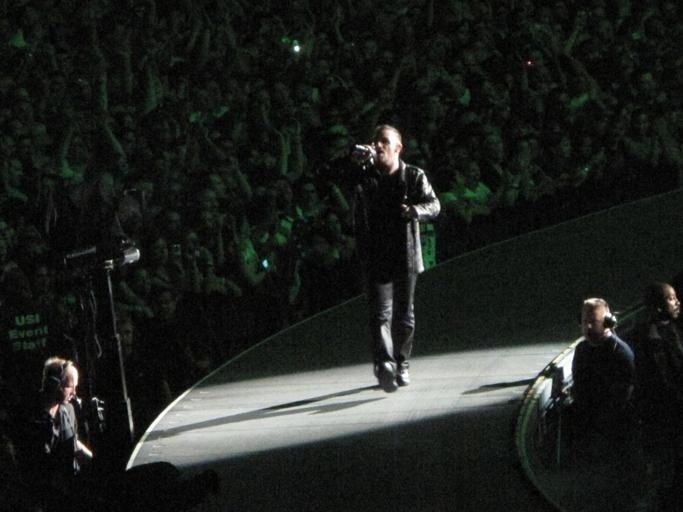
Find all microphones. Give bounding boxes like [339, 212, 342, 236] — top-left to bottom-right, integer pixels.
[610, 328, 618, 351]
[354, 143, 379, 156]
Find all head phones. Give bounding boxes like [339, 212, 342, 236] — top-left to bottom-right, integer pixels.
[576, 297, 616, 328]
[43, 360, 74, 395]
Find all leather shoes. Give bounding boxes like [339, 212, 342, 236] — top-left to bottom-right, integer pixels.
[374, 361, 411, 393]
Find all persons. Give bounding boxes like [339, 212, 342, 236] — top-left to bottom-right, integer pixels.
[347, 123, 443, 393]
[550, 281, 683, 512]
[343, 1, 682, 281]
[1, 1, 343, 511]
